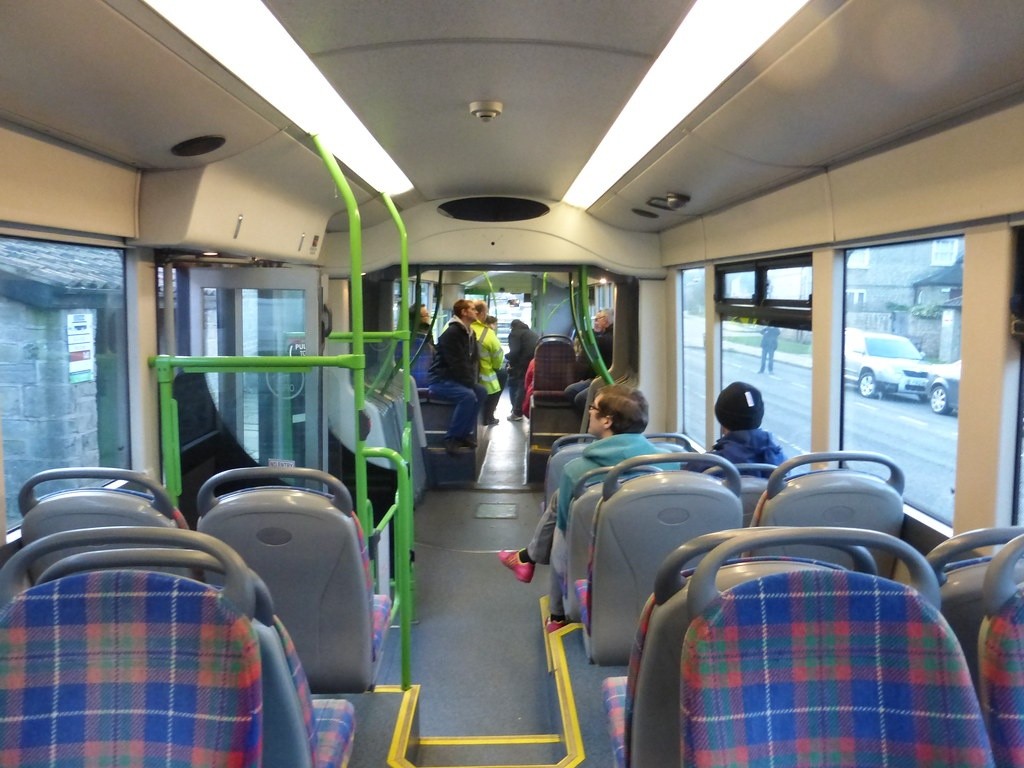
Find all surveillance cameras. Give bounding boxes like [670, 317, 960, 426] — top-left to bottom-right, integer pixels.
[666, 193, 690, 209]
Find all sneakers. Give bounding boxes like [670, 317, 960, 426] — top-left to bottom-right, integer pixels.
[546, 616, 565, 633]
[499, 548, 535, 583]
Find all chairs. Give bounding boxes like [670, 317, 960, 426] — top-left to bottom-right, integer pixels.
[533, 335, 577, 397]
[396, 331, 437, 396]
[545, 433, 1024, 768]
[0, 466, 393, 768]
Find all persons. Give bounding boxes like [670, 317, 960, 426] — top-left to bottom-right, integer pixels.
[758, 326, 780, 373]
[498, 381, 787, 633]
[409, 300, 539, 450]
[564, 309, 613, 412]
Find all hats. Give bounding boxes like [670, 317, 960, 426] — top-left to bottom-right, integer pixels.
[715, 382, 764, 431]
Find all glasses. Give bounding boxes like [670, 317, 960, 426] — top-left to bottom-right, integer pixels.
[595, 316, 605, 321]
[589, 402, 599, 411]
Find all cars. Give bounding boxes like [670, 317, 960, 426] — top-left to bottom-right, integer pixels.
[487, 298, 522, 342]
[843, 327, 931, 404]
[926, 361, 961, 414]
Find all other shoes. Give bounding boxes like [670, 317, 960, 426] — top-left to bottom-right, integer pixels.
[507, 412, 523, 421]
[442, 440, 456, 453]
[459, 438, 478, 447]
[487, 419, 499, 424]
